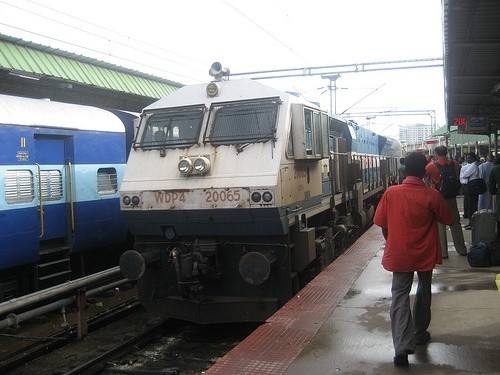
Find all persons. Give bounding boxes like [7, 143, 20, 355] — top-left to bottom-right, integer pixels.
[398, 158, 406, 184]
[373, 152, 454, 366]
[424, 146, 467, 258]
[450, 152, 500, 229]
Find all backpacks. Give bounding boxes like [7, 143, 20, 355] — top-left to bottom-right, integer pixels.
[434, 161, 462, 197]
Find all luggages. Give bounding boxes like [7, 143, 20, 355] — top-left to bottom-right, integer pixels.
[471, 208, 496, 244]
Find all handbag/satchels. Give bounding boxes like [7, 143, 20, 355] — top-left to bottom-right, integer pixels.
[466, 241, 499, 267]
[461, 179, 487, 195]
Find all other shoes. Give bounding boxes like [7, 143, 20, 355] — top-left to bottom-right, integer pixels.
[464, 224, 472, 230]
[393, 354, 409, 365]
[414, 332, 430, 344]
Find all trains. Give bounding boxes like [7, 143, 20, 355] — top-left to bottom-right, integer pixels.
[0, 93, 128, 314]
[119, 62, 408, 324]
